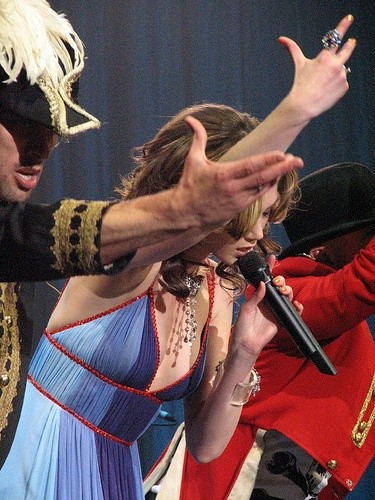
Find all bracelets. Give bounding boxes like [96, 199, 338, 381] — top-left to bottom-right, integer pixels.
[212, 358, 261, 406]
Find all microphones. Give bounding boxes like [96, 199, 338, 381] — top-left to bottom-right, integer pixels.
[236, 250, 337, 376]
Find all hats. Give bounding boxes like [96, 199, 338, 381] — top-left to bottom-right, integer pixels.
[277, 162, 375, 261]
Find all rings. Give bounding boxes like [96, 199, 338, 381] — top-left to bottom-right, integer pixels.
[321, 29, 342, 50]
[345, 67, 352, 76]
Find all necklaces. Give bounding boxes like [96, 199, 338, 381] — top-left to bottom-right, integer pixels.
[181, 275, 205, 355]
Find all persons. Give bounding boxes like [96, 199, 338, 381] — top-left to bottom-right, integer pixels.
[141, 162, 375, 500]
[0, 15, 355, 500]
[0, 0, 303, 471]
